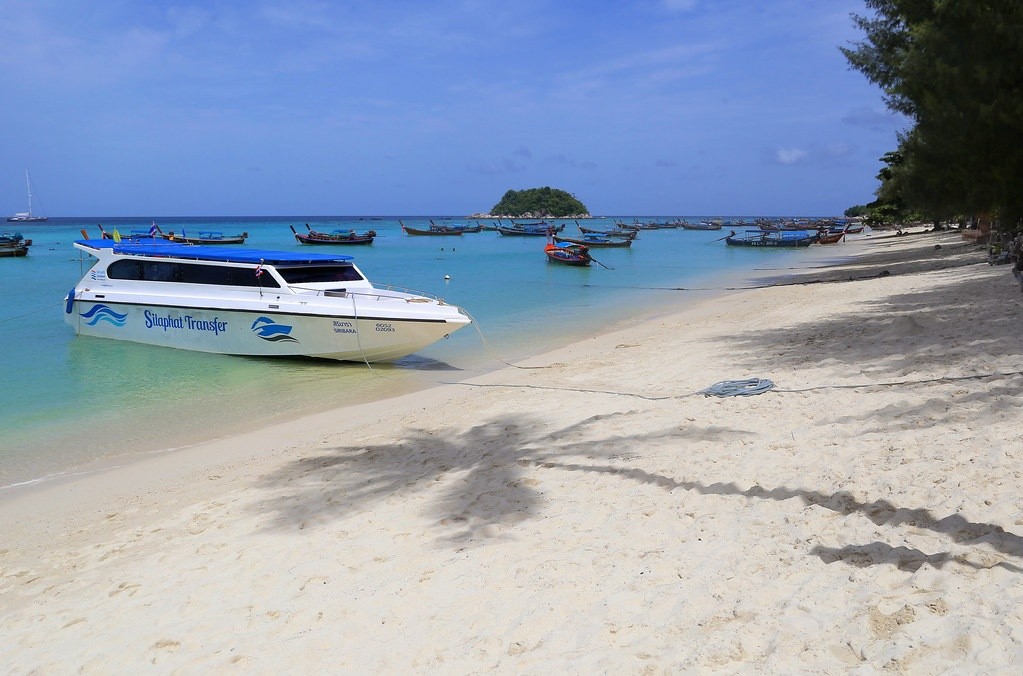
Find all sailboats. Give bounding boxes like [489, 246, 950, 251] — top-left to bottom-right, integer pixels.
[7, 171, 47, 221]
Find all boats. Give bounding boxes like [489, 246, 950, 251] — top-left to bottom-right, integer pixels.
[98, 224, 248, 245]
[65, 221, 473, 364]
[544, 220, 639, 267]
[400, 219, 566, 236]
[290, 224, 377, 244]
[615, 216, 863, 247]
[0, 232, 32, 257]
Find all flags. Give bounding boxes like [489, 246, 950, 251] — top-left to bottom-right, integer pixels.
[147, 221, 157, 237]
[255, 266, 260, 277]
